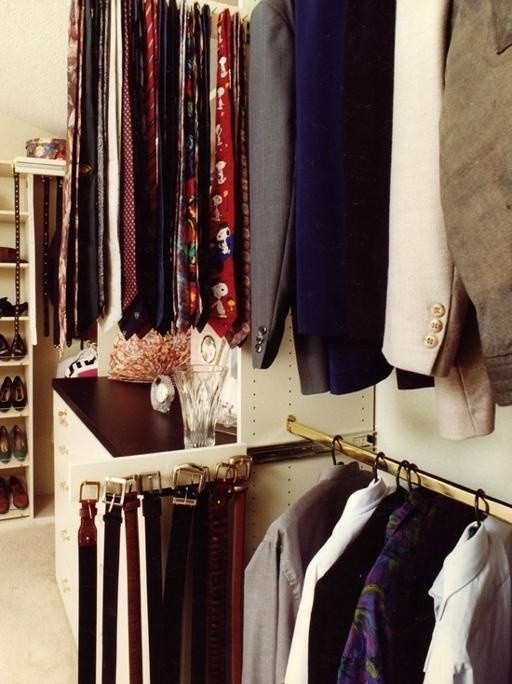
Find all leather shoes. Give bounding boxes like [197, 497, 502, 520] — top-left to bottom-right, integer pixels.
[1, 332, 30, 517]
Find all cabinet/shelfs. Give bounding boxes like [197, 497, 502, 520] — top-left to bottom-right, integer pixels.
[52, 375, 237, 654]
[0, 159, 35, 519]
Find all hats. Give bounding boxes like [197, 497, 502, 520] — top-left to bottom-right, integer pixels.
[0, 247, 28, 263]
